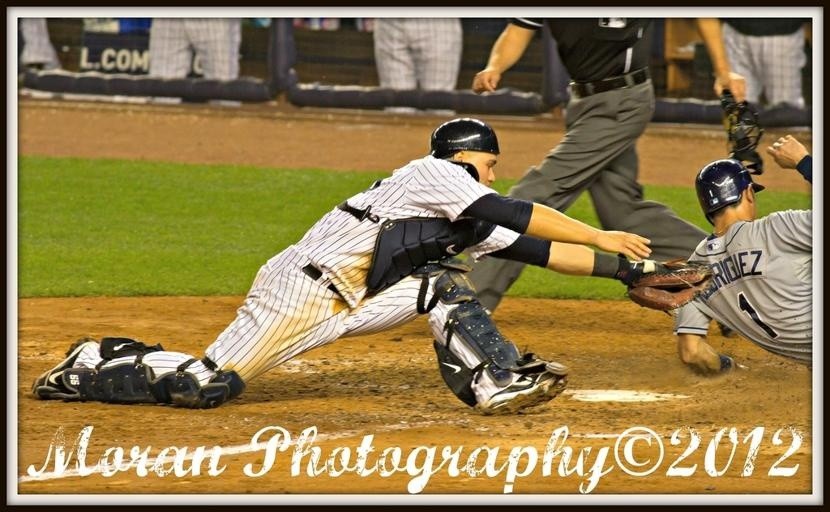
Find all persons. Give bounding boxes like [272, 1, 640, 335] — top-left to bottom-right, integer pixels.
[373, 18, 463, 116]
[455, 17, 748, 314]
[30, 117, 715, 416]
[721, 18, 809, 109]
[674, 133, 812, 377]
[147, 18, 244, 107]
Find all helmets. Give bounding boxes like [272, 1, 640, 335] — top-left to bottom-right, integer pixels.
[695, 159, 765, 225]
[432, 118, 500, 160]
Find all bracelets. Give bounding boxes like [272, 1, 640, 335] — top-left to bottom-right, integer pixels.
[716, 354, 732, 374]
[796, 156, 812, 184]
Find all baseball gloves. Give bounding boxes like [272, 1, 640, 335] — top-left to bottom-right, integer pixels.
[617, 257, 717, 309]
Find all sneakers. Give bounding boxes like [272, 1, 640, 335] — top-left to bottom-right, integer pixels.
[469, 370, 567, 416]
[30, 338, 100, 400]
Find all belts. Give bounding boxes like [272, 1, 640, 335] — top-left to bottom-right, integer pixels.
[568, 65, 648, 97]
[301, 264, 342, 300]
